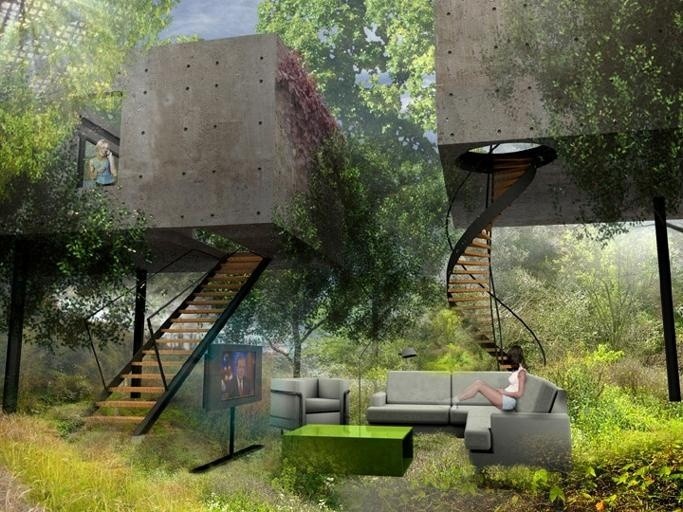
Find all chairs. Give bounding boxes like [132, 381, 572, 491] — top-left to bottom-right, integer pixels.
[266, 374, 353, 438]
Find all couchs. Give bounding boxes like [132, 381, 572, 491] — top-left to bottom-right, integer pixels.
[461, 372, 574, 488]
[362, 368, 516, 427]
